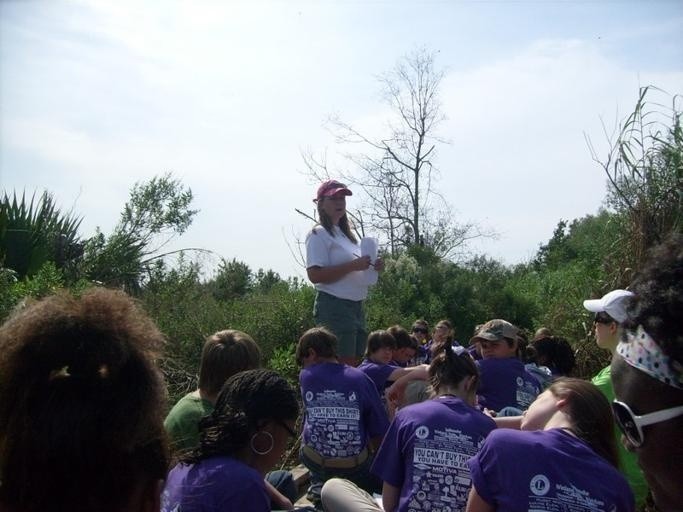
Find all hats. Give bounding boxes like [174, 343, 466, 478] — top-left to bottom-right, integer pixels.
[317, 179, 352, 200]
[468, 318, 517, 347]
[582, 288, 642, 328]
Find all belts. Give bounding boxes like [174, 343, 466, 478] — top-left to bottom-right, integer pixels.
[302, 444, 369, 469]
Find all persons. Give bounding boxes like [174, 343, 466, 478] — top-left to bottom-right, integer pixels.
[305, 181, 385, 365]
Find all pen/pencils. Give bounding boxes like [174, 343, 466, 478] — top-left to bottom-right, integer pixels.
[352, 252, 377, 267]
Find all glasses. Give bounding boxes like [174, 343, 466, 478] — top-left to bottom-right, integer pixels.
[610, 397, 683, 445]
[591, 312, 613, 326]
[413, 327, 428, 334]
